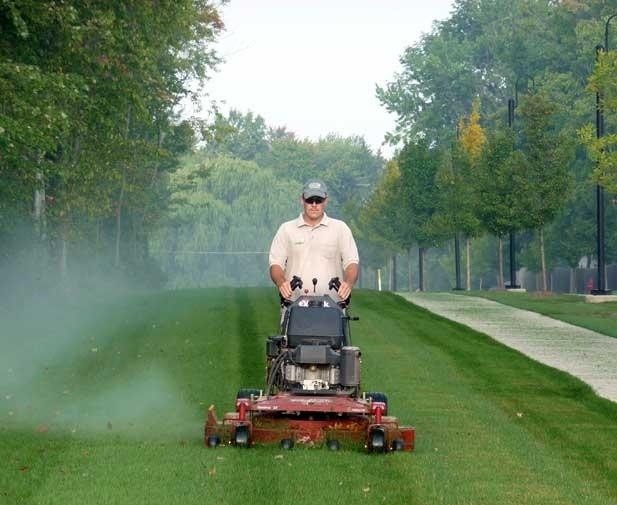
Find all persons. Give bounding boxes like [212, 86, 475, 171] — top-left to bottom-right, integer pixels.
[585, 276, 594, 294]
[269, 179, 359, 317]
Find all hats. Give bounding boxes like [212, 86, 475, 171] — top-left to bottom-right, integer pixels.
[302, 180, 327, 200]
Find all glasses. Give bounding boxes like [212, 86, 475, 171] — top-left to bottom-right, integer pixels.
[304, 198, 325, 203]
